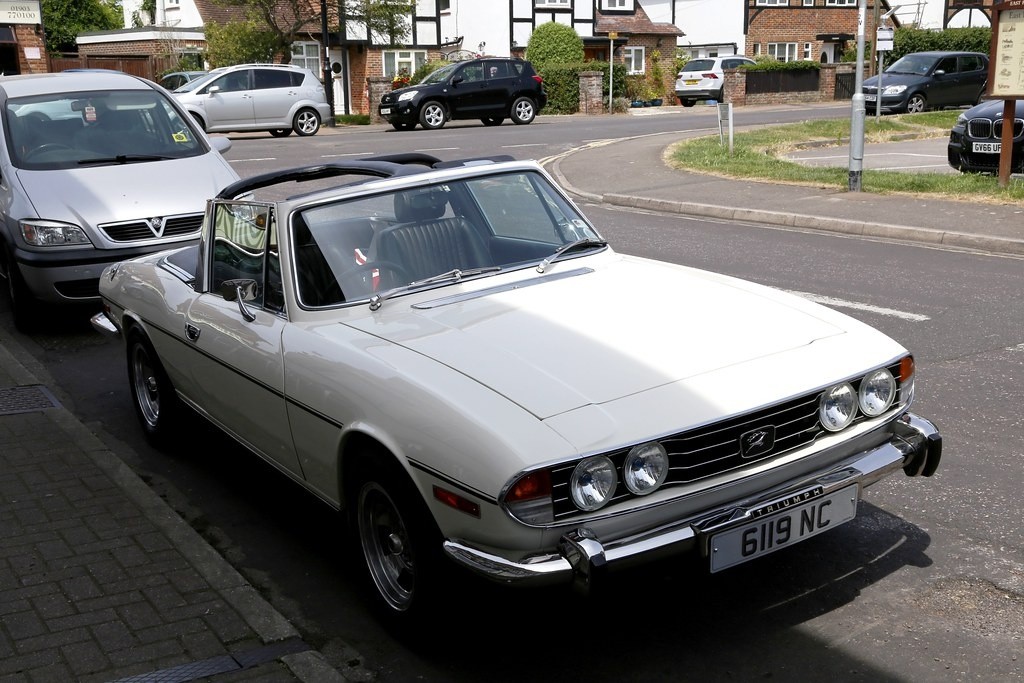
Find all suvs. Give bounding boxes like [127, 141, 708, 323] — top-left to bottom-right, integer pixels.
[377, 57, 548, 131]
[860, 51, 990, 116]
[674, 56, 758, 107]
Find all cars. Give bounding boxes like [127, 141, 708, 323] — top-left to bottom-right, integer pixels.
[947, 99, 1024, 176]
[145, 64, 332, 151]
[14, 69, 247, 125]
[0, 73, 259, 337]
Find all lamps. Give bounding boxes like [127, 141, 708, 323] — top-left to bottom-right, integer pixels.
[657, 37, 662, 47]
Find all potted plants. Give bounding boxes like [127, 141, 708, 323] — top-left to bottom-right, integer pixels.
[629, 49, 690, 107]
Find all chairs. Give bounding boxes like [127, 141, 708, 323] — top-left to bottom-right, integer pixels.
[367, 185, 495, 291]
[475, 71, 483, 81]
[81, 109, 157, 157]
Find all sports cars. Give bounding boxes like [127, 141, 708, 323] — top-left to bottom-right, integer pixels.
[89, 155, 942, 660]
